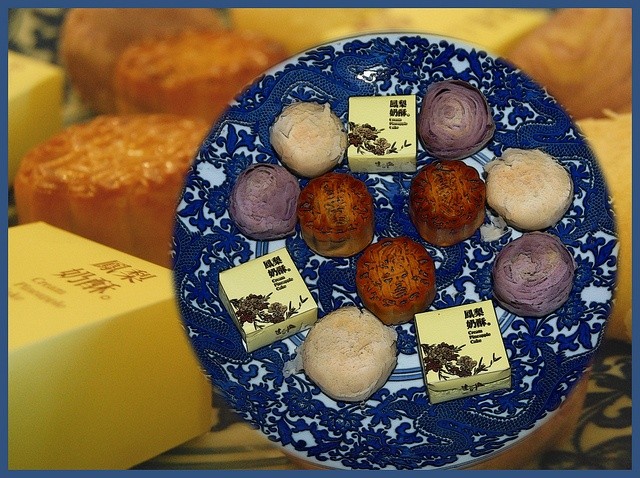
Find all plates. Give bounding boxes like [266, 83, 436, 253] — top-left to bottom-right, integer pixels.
[166, 30, 621, 472]
